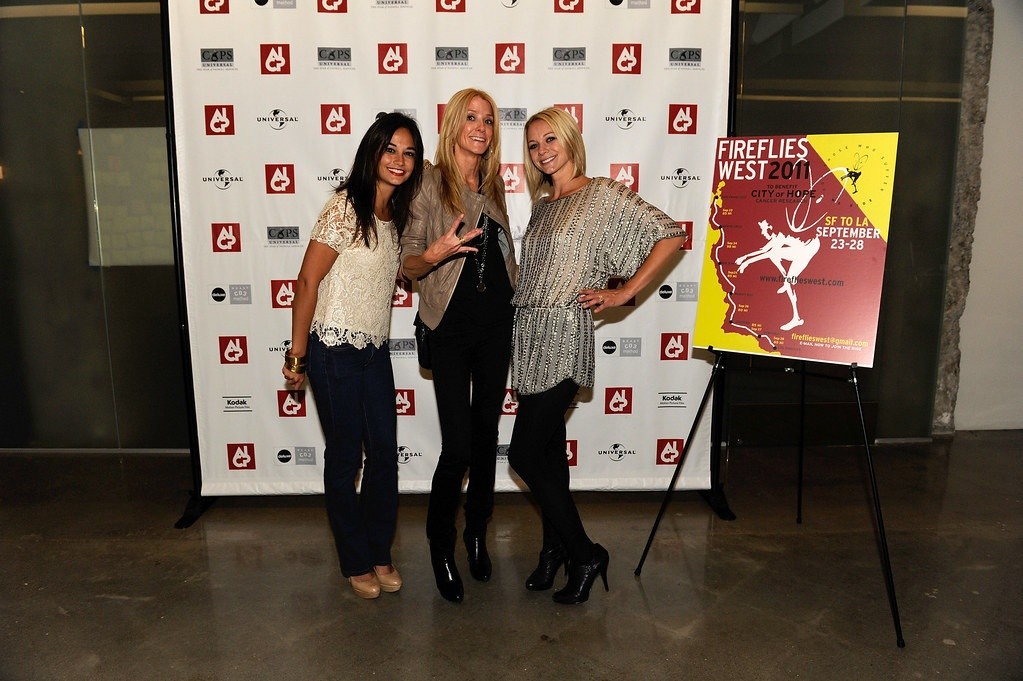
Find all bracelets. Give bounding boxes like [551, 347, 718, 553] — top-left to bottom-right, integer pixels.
[284, 349, 306, 374]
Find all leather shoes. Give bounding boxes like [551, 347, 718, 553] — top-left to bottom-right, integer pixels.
[430, 548, 464, 603]
[463, 527, 492, 582]
[348, 572, 380, 598]
[373, 563, 403, 592]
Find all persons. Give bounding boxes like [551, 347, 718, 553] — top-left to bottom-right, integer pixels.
[400, 88, 516, 601]
[513, 109, 688, 605]
[280, 113, 423, 598]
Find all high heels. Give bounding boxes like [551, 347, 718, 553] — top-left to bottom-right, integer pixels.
[551, 543, 610, 605]
[525, 547, 570, 592]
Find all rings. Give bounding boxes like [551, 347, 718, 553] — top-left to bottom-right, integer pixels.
[593, 288, 599, 293]
[459, 237, 465, 244]
[600, 297, 604, 304]
